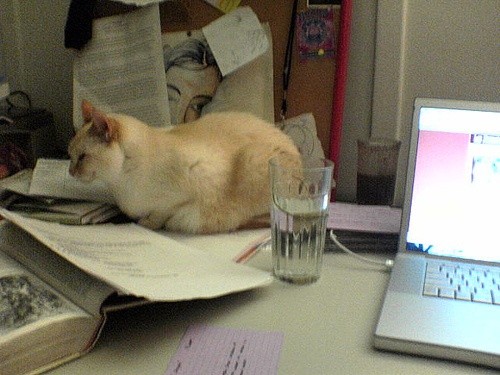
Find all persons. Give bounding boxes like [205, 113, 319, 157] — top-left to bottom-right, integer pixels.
[164, 37, 227, 127]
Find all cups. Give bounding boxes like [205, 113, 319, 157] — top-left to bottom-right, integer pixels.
[268, 153, 335, 286]
[356, 136, 401, 206]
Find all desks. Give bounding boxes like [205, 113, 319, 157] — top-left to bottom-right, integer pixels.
[0, 122, 56, 168]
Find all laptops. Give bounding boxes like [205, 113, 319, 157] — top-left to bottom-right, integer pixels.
[373, 95, 500, 369]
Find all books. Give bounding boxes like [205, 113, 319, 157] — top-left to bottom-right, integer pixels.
[0, 217, 153, 375]
[0, 202, 122, 225]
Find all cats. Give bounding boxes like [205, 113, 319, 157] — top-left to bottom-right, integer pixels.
[68, 98, 315, 235]
